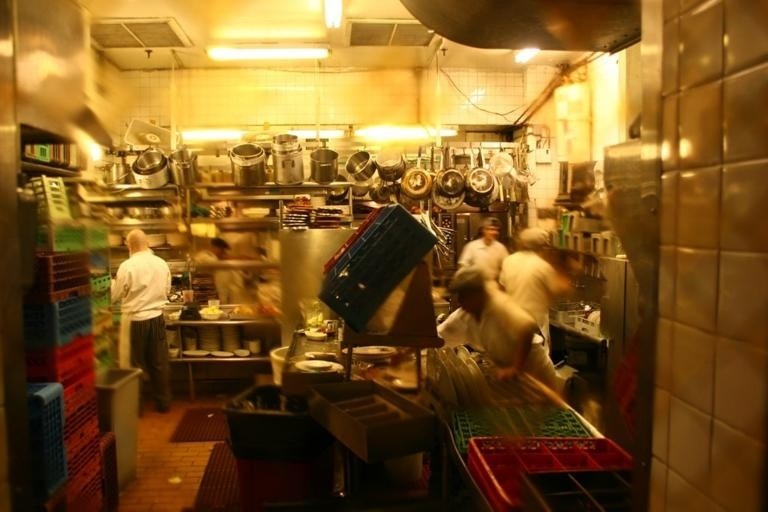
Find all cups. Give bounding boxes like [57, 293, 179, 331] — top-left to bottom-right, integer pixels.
[321, 320, 340, 337]
[168, 348, 179, 357]
[169, 314, 180, 320]
[182, 290, 194, 303]
[557, 212, 626, 260]
[300, 299, 324, 332]
[207, 300, 220, 309]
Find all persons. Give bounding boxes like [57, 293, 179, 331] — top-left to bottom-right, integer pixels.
[396, 265, 558, 392]
[111, 229, 174, 412]
[211, 214, 261, 303]
[500, 229, 571, 361]
[459, 217, 510, 280]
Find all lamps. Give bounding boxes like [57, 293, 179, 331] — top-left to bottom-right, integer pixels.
[207, 44, 330, 64]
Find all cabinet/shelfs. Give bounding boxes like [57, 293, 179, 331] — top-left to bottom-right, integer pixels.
[177, 182, 356, 309]
[541, 240, 608, 347]
[87, 181, 186, 321]
[163, 316, 281, 396]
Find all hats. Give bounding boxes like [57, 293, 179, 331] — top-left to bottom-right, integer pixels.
[517, 228, 550, 248]
[447, 266, 485, 293]
[480, 218, 502, 225]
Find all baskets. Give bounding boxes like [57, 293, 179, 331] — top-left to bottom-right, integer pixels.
[28, 174, 118, 511]
[452, 408, 652, 511]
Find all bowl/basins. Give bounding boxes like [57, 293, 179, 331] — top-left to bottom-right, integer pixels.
[106, 206, 184, 220]
[198, 310, 223, 320]
[304, 330, 326, 340]
[241, 208, 270, 217]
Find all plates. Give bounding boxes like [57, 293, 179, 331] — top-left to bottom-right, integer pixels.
[183, 325, 260, 358]
[109, 232, 185, 247]
[294, 345, 397, 376]
[426, 344, 489, 403]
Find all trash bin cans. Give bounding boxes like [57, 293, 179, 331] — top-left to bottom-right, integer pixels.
[220, 382, 338, 511]
[94, 366, 144, 494]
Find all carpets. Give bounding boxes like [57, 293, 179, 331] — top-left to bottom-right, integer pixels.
[167, 405, 231, 442]
[188, 440, 340, 511]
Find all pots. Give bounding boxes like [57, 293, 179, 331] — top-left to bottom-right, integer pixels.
[131, 132, 516, 210]
